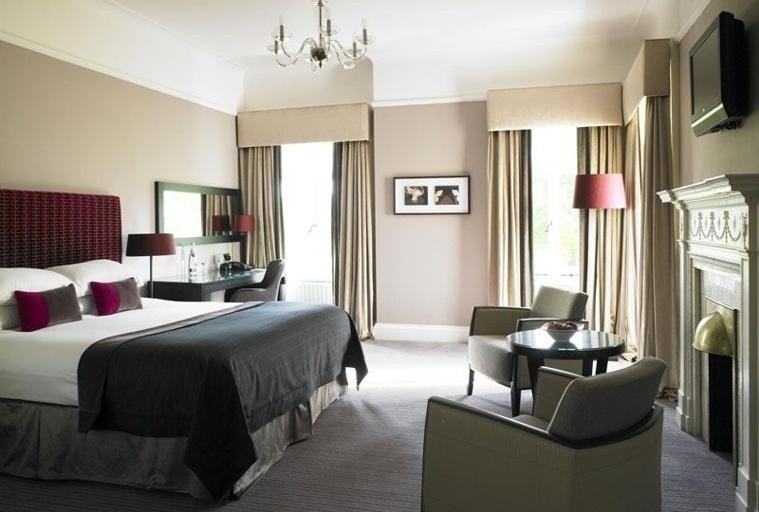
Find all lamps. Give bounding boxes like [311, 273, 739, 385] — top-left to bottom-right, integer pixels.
[212, 215, 232, 235]
[125, 232, 177, 298]
[187, 241, 196, 279]
[573, 170, 628, 330]
[267, 1, 376, 71]
[233, 214, 255, 271]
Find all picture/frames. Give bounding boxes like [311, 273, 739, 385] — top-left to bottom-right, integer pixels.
[392, 175, 472, 215]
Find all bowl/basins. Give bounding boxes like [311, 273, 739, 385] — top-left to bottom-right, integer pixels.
[549, 341, 577, 349]
[544, 326, 582, 341]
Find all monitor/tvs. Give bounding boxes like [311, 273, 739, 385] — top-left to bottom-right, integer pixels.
[687, 10, 752, 137]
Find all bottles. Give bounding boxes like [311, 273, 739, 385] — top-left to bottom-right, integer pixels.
[200, 262, 207, 275]
[188, 243, 200, 276]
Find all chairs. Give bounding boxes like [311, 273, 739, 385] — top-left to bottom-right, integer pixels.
[467, 286, 589, 416]
[224, 259, 285, 302]
[420, 355, 667, 511]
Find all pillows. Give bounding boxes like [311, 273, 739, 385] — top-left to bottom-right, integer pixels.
[0, 267, 86, 307]
[90, 277, 143, 315]
[0, 302, 83, 330]
[15, 284, 83, 332]
[44, 258, 144, 297]
[78, 295, 98, 314]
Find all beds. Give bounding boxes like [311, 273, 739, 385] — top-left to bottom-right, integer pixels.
[0, 187, 369, 507]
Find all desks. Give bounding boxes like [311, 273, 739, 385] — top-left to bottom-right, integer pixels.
[147, 268, 268, 301]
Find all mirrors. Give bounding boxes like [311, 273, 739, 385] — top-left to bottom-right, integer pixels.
[154, 181, 244, 246]
